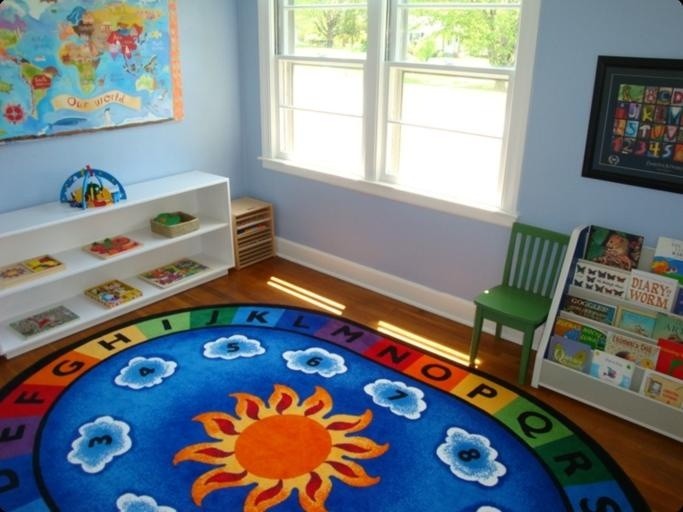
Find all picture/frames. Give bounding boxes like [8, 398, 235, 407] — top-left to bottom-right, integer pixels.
[574, 53, 682, 195]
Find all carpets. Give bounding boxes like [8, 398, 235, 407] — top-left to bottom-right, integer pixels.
[0, 303, 655, 511]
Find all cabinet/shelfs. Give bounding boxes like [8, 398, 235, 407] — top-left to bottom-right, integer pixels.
[533, 220, 682, 447]
[225, 194, 277, 271]
[1, 171, 236, 364]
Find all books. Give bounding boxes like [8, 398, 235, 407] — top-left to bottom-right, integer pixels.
[541, 224, 683, 412]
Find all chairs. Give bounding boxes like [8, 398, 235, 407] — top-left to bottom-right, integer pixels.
[468, 220, 572, 379]
